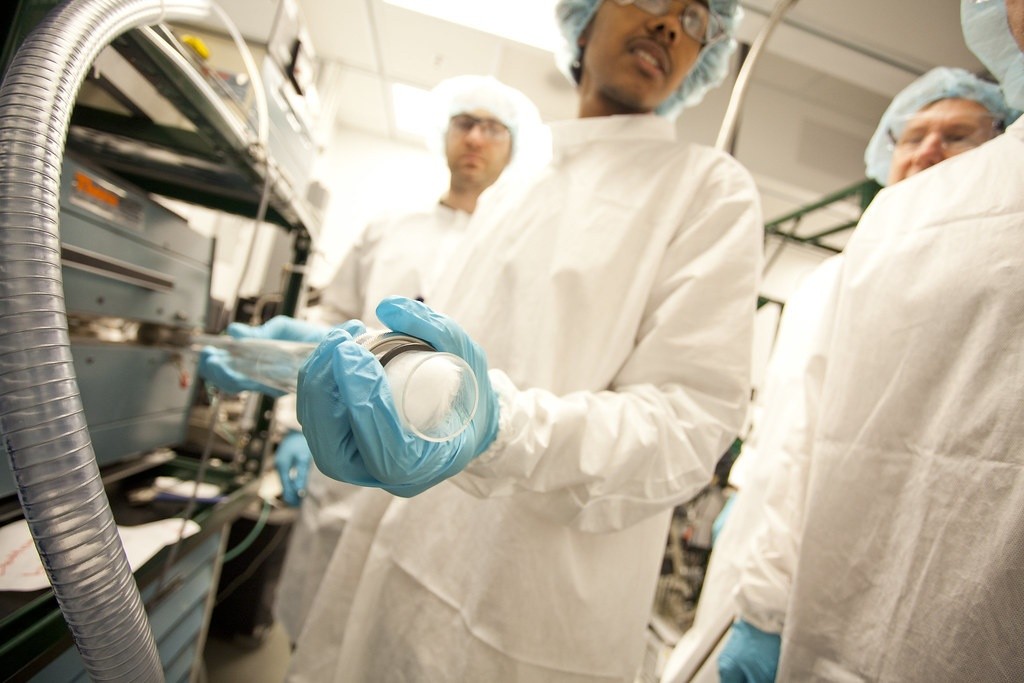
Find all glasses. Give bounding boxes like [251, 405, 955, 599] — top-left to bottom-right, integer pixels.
[888, 115, 997, 150]
[618, 2, 722, 45]
[449, 115, 508, 142]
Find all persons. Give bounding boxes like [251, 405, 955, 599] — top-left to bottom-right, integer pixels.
[273, 79, 542, 648]
[660, 0, 1024, 683]
[202, 1, 764, 682]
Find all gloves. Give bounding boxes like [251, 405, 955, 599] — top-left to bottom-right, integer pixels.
[714, 621, 781, 683]
[272, 430, 318, 507]
[198, 314, 332, 400]
[295, 295, 497, 500]
